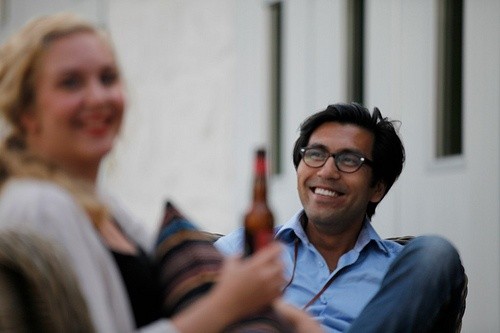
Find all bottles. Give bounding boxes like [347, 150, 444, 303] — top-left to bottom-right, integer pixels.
[243, 146, 274, 258]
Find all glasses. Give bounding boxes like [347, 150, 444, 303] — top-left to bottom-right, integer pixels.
[298, 147, 374, 173]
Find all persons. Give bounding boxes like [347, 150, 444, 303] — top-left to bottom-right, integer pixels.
[214, 100, 469, 333]
[0, 10, 321, 333]
